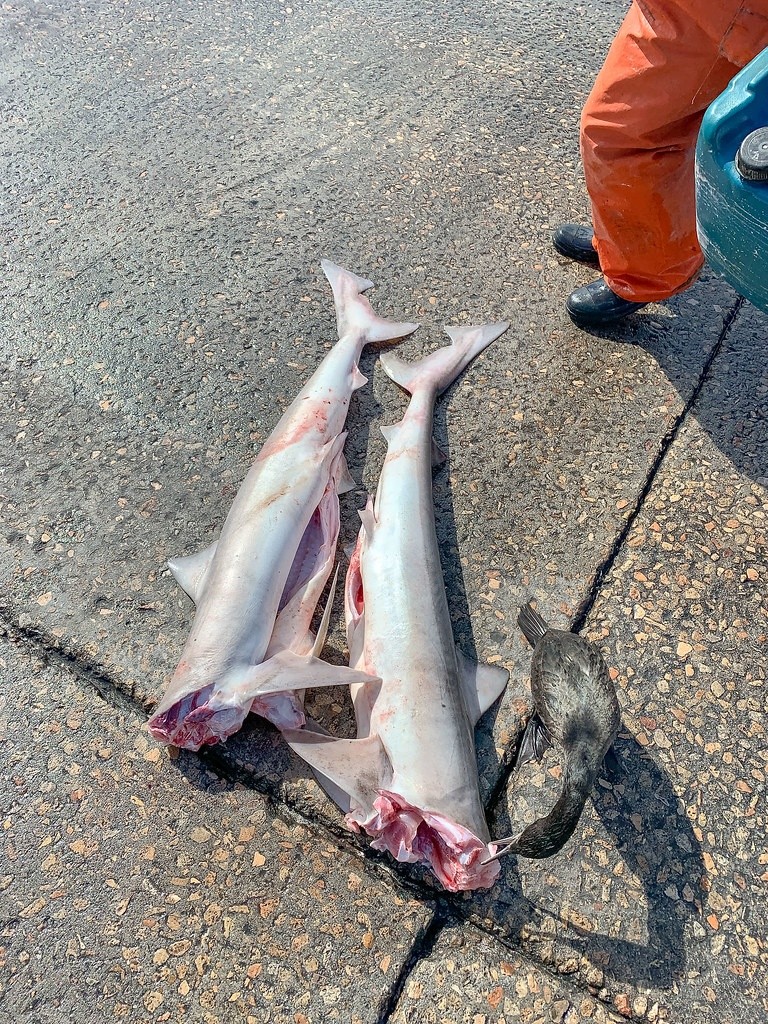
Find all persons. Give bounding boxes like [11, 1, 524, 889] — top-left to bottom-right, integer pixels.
[555, 0, 768, 322]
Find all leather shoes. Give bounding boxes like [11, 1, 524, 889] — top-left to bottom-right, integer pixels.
[553, 224, 600, 263]
[565, 277, 650, 323]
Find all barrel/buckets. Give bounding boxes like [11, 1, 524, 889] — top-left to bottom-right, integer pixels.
[694, 46, 768, 315]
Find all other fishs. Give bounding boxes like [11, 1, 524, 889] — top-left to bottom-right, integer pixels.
[281, 318, 500, 894]
[143, 260, 422, 752]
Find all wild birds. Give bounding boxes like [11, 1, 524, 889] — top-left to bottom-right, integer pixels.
[480, 599, 630, 868]
